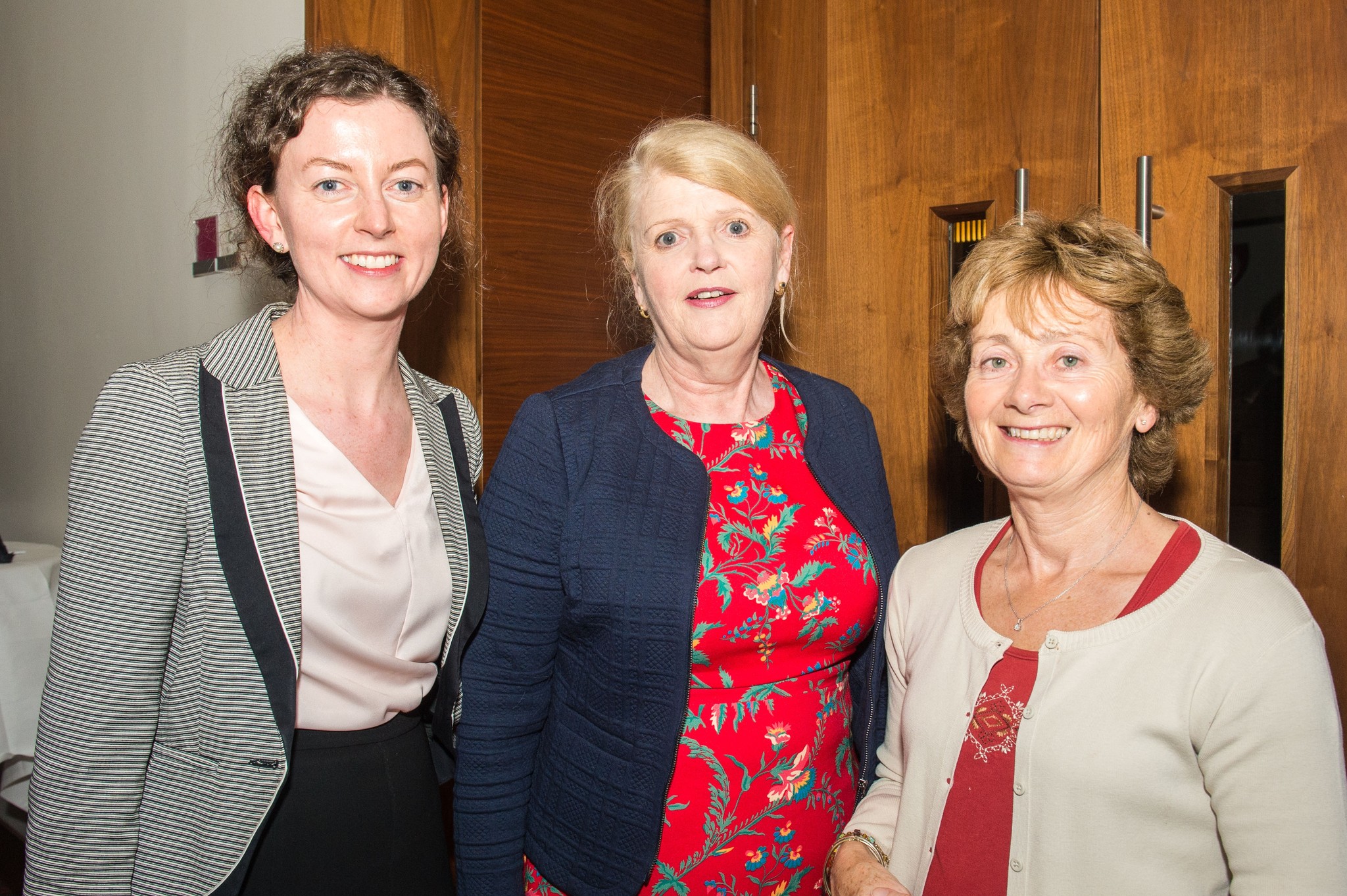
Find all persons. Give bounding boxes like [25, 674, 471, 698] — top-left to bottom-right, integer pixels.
[22, 45, 490, 896]
[822, 208, 1347, 896]
[450, 116, 899, 896]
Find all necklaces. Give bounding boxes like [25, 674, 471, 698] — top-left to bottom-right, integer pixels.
[1002, 497, 1145, 632]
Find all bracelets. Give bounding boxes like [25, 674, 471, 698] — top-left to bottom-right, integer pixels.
[825, 831, 890, 896]
